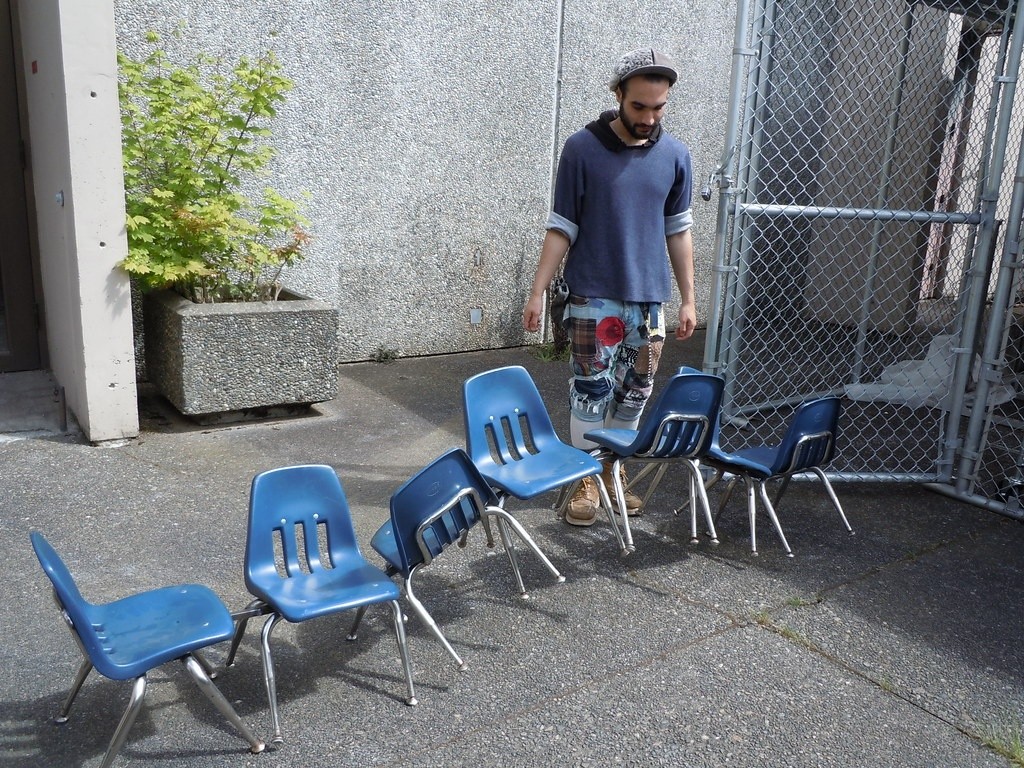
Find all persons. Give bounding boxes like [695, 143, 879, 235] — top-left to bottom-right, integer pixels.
[522, 48, 697, 526]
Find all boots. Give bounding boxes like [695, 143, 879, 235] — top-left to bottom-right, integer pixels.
[600, 462, 644, 515]
[566, 454, 600, 525]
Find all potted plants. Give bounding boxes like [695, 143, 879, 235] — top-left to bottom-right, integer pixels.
[114, 30, 340, 417]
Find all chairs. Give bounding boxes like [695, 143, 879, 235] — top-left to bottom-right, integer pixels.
[343, 448, 565, 670]
[595, 366, 773, 559]
[224, 461, 419, 744]
[707, 397, 856, 559]
[30, 532, 266, 767]
[554, 373, 724, 552]
[455, 361, 629, 599]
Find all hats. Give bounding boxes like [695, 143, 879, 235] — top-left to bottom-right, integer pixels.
[608, 47, 678, 91]
